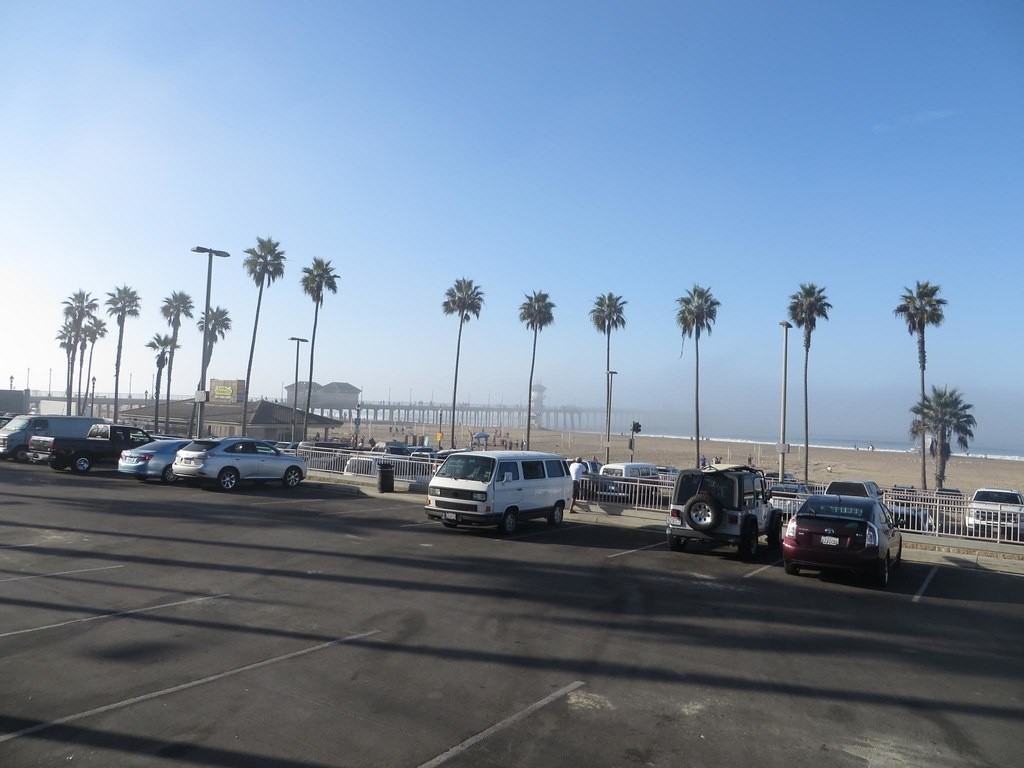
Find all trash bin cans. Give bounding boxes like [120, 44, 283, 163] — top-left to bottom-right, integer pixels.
[375, 462, 396, 493]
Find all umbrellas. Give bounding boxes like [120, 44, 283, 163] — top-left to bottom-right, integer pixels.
[472, 432, 489, 438]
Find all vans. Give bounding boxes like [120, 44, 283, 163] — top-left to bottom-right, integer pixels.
[423, 451, 574, 538]
[0, 414, 105, 463]
[567, 459, 679, 505]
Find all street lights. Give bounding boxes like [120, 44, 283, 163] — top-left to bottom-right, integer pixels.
[91, 376, 96, 417]
[288, 337, 309, 441]
[777, 320, 794, 483]
[190, 246, 231, 438]
[605, 370, 618, 461]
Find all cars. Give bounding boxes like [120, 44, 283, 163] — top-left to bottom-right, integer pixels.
[117, 439, 191, 485]
[262, 440, 464, 477]
[782, 494, 903, 589]
[0, 416, 14, 430]
[171, 437, 308, 491]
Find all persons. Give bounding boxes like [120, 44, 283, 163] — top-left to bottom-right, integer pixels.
[700, 455, 706, 467]
[747, 454, 753, 465]
[827, 465, 831, 473]
[468, 428, 526, 451]
[316, 431, 319, 441]
[389, 426, 404, 437]
[350, 435, 376, 452]
[592, 456, 597, 461]
[712, 456, 722, 465]
[569, 456, 587, 513]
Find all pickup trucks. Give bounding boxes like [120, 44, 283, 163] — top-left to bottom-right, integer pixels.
[25, 424, 152, 474]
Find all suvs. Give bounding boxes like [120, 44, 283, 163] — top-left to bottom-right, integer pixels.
[665, 468, 783, 561]
[765, 472, 1024, 539]
